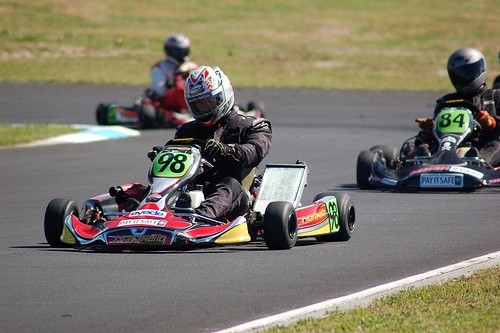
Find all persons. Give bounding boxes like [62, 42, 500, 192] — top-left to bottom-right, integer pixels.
[492, 50, 500, 90]
[404, 47, 500, 166]
[114, 66, 273, 225]
[151, 34, 191, 97]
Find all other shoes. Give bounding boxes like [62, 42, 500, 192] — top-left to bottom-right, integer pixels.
[124, 198, 139, 212]
[464, 148, 481, 166]
[416, 145, 431, 158]
[174, 193, 191, 220]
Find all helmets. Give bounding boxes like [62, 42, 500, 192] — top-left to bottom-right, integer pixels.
[164, 35, 191, 65]
[447, 48, 488, 93]
[184, 66, 234, 126]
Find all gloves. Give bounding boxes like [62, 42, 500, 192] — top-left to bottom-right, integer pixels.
[415, 118, 435, 131]
[480, 111, 496, 127]
[148, 146, 163, 161]
[201, 139, 233, 159]
